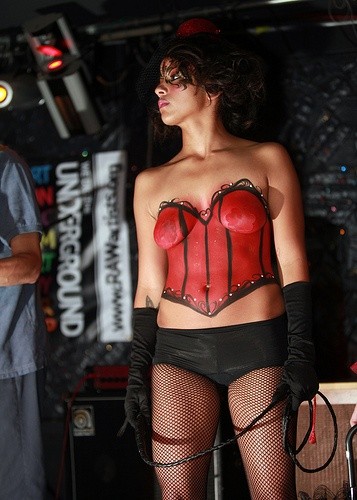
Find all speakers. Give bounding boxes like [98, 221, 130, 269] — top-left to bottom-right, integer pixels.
[65, 389, 156, 500]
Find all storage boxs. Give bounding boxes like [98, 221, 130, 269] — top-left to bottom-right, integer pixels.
[64, 396, 222, 500]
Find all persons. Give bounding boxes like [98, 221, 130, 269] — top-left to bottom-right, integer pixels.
[124, 30, 321, 500]
[0, 142, 49, 500]
